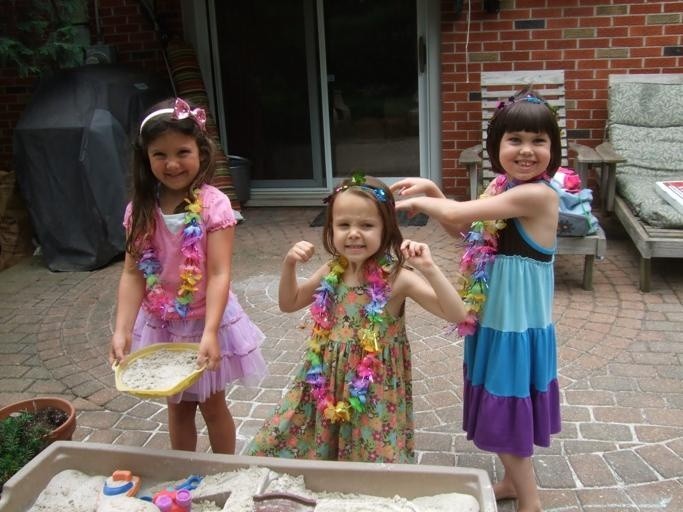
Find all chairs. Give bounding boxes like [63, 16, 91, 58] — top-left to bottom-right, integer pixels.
[458, 70, 606, 291]
[596, 73, 683, 292]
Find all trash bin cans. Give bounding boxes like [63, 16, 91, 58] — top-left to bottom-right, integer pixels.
[229, 154, 252, 203]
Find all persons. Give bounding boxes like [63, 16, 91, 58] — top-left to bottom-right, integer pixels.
[108, 98, 269, 454]
[251, 177, 468, 463]
[387, 90, 561, 512]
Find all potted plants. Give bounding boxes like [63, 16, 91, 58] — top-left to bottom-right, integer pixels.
[1, 397, 74, 449]
